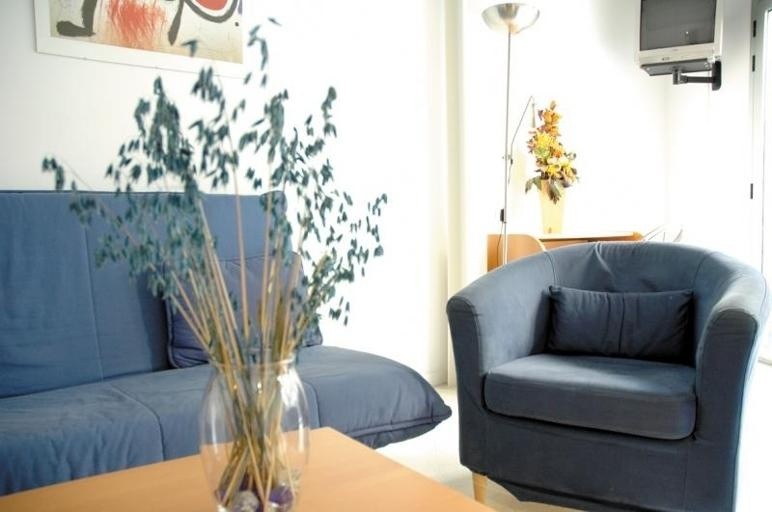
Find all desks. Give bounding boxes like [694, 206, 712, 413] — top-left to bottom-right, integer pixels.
[538, 232, 646, 250]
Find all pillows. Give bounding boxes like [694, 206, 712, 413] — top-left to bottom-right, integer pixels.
[160, 252, 324, 369]
[549, 285, 695, 366]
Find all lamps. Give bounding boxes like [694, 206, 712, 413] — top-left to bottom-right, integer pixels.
[481, 2, 539, 268]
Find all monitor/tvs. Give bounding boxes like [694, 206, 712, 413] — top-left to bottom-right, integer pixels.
[633, 1, 723, 65]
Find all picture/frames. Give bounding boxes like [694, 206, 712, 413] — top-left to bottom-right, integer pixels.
[32, 0, 249, 79]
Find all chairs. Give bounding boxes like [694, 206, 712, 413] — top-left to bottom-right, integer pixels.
[484, 232, 545, 276]
[447, 237, 767, 512]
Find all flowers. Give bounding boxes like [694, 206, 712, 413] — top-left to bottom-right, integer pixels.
[525, 98, 580, 203]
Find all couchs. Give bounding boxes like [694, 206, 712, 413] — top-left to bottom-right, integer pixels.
[2, 188, 451, 493]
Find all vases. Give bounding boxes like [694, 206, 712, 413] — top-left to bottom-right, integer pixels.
[198, 350, 311, 511]
[539, 179, 563, 237]
